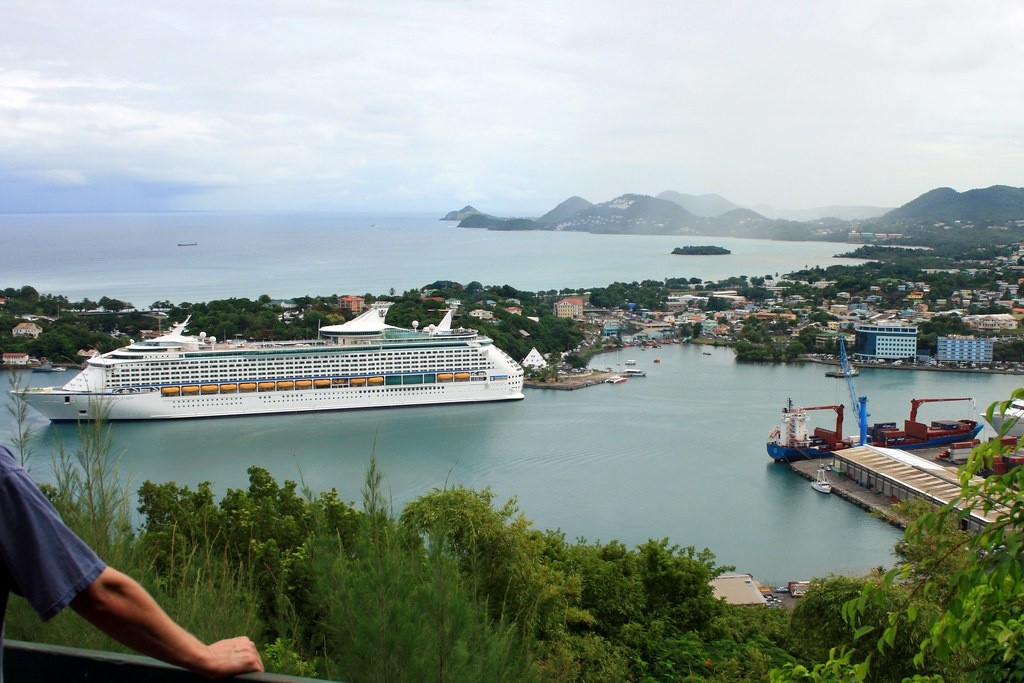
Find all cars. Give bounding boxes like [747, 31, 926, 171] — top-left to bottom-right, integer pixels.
[553, 319, 604, 378]
[804, 352, 1024, 373]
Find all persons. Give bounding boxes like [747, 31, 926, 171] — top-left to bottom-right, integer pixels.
[0, 445, 265, 683]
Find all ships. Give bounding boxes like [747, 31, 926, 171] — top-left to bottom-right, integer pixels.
[8, 299, 525, 424]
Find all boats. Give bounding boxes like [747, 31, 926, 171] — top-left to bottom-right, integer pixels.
[258, 382, 275, 390]
[980, 397, 1024, 438]
[161, 386, 180, 395]
[30, 360, 67, 373]
[810, 469, 832, 494]
[437, 373, 453, 381]
[295, 380, 312, 389]
[239, 383, 256, 391]
[824, 363, 861, 378]
[624, 368, 647, 377]
[766, 419, 985, 463]
[367, 377, 384, 384]
[604, 374, 628, 384]
[182, 385, 199, 394]
[455, 372, 470, 380]
[277, 381, 294, 390]
[220, 383, 237, 393]
[201, 384, 218, 393]
[313, 380, 330, 386]
[350, 378, 366, 385]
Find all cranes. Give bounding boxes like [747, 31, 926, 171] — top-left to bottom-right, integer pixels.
[839, 332, 871, 448]
